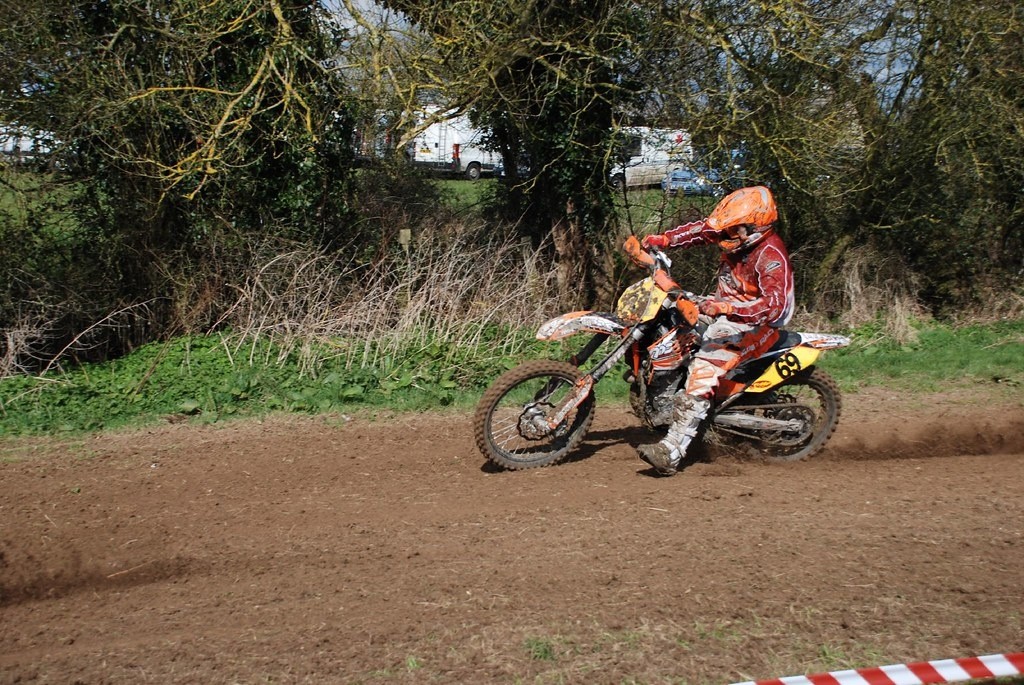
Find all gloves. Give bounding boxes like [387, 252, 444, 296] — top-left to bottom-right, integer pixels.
[642, 233, 671, 251]
[698, 301, 729, 317]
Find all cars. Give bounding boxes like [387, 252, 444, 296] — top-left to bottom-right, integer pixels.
[661, 150, 774, 197]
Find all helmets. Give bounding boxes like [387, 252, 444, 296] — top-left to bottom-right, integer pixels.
[705, 186, 777, 254]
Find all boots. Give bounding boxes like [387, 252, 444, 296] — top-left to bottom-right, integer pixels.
[622, 370, 635, 382]
[637, 390, 711, 475]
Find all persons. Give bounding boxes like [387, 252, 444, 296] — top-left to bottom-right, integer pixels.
[636, 186, 795, 476]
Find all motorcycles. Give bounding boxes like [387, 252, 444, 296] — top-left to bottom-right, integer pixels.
[472, 235, 851, 471]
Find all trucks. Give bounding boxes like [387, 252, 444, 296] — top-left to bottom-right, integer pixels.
[326, 104, 504, 183]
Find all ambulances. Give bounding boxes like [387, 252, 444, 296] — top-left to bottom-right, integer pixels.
[608, 127, 693, 191]
